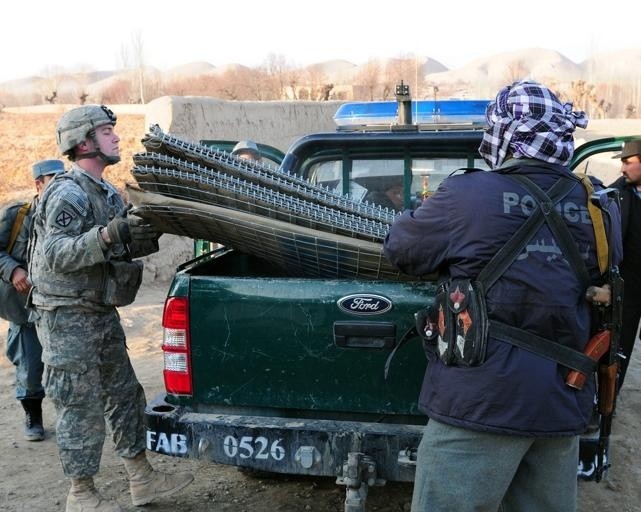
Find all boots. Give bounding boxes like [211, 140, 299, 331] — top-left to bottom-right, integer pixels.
[123, 449, 198, 504]
[64, 476, 129, 512]
[20, 397, 45, 442]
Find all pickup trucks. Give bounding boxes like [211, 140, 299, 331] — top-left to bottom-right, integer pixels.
[143, 82, 640, 487]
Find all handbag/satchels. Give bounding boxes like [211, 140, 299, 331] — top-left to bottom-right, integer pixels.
[0, 278, 35, 330]
[433, 280, 489, 368]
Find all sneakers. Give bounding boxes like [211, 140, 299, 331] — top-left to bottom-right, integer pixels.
[573, 443, 612, 481]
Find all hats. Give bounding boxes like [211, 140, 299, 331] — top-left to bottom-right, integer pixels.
[611, 135, 641, 159]
[31, 158, 67, 180]
[230, 139, 263, 160]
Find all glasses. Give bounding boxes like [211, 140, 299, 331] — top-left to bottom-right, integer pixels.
[56, 103, 117, 155]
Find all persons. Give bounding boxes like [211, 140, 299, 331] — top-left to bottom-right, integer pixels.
[0, 160, 65, 441]
[26, 103, 195, 511]
[366, 175, 404, 210]
[381, 76, 626, 512]
[209, 139, 263, 258]
[607, 137, 641, 417]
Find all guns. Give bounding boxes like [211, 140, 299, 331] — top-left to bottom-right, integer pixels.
[565, 265, 625, 482]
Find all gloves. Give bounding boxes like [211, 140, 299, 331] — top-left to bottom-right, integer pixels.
[106, 204, 157, 242]
